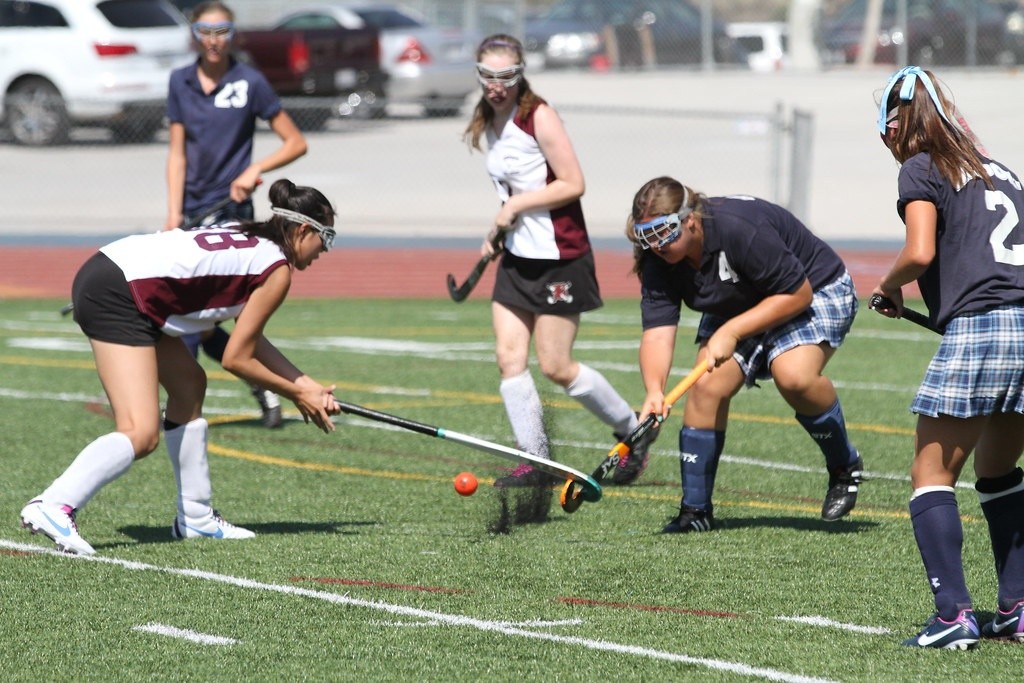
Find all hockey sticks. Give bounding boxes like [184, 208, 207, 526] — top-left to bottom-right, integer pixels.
[872, 295, 945, 336]
[334, 399, 603, 503]
[559, 358, 708, 513]
[59, 178, 264, 316]
[447, 216, 518, 303]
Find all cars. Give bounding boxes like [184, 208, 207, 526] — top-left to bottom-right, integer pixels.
[519, 1, 765, 72]
[825, 0, 1024, 67]
[239, 8, 469, 133]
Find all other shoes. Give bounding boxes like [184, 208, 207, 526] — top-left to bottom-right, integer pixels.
[262, 406, 285, 430]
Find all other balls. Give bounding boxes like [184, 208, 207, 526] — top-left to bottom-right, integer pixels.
[454, 472, 478, 496]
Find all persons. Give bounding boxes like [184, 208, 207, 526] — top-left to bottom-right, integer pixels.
[164, 0, 307, 428]
[625, 176, 863, 537]
[868, 67, 1023, 652]
[20, 176, 339, 558]
[460, 33, 652, 488]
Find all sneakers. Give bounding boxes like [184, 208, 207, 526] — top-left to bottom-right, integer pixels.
[659, 504, 715, 534]
[20, 494, 97, 558]
[494, 462, 553, 487]
[171, 509, 257, 544]
[901, 608, 979, 651]
[612, 412, 661, 485]
[820, 450, 865, 521]
[982, 602, 1024, 644]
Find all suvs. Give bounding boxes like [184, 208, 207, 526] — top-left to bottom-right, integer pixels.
[0, 0, 203, 149]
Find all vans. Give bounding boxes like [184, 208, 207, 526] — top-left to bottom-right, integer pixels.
[722, 21, 790, 72]
